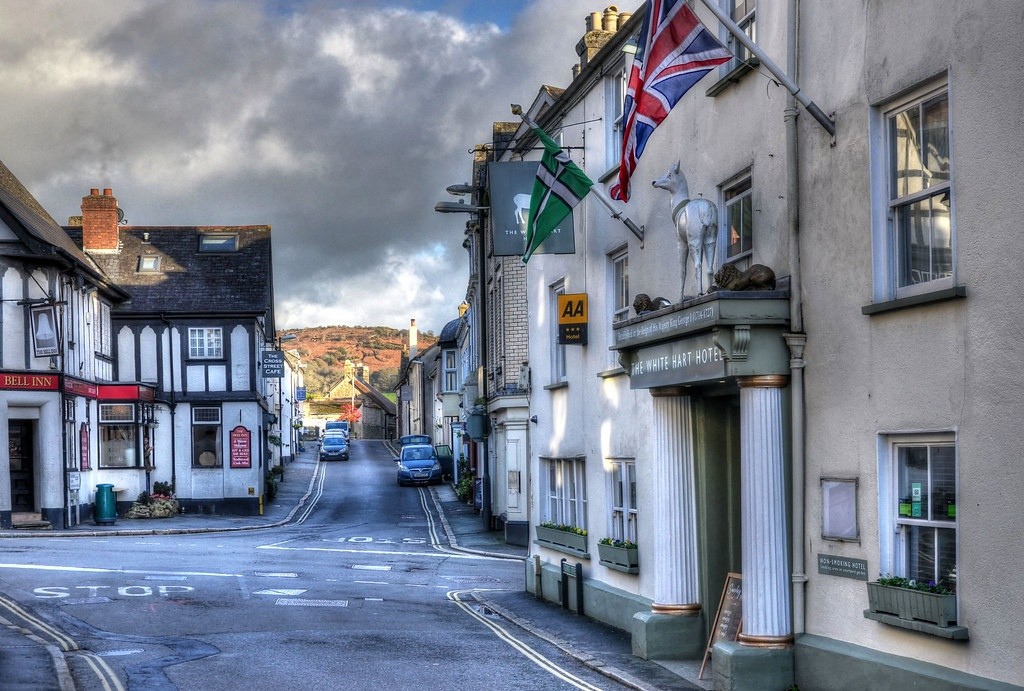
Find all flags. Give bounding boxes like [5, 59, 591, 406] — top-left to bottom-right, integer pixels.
[522, 127, 595, 264]
[607, 0, 736, 203]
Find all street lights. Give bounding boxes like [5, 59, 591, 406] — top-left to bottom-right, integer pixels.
[447, 185, 492, 531]
[277, 334, 296, 483]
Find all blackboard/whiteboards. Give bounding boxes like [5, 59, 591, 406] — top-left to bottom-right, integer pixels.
[472, 476, 482, 510]
[706, 571, 745, 651]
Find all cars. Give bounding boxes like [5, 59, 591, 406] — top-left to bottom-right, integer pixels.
[317, 421, 352, 461]
[393, 434, 454, 486]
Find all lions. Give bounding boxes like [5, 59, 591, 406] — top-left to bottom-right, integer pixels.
[713, 262, 777, 291]
[633, 293, 672, 315]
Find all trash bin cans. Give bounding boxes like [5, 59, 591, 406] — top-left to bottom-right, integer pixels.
[94, 483, 117, 525]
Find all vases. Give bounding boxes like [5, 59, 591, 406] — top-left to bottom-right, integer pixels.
[535, 525, 587, 553]
[866, 581, 957, 627]
[597, 543, 638, 568]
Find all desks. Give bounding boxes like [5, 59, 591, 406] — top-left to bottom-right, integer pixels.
[93, 488, 126, 517]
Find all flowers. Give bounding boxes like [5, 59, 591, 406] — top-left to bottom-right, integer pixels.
[598, 537, 637, 549]
[876, 572, 951, 594]
[539, 520, 587, 536]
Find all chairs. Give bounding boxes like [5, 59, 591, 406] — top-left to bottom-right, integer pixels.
[405, 453, 415, 460]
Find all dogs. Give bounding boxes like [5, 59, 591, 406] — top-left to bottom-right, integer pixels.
[651, 158, 719, 305]
